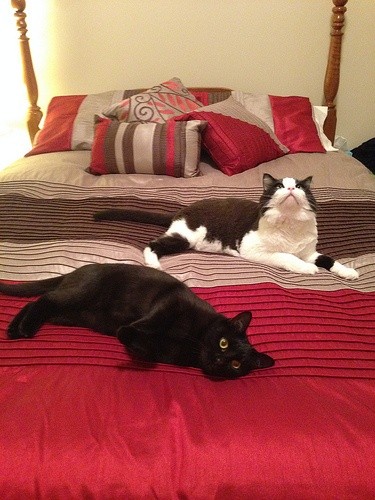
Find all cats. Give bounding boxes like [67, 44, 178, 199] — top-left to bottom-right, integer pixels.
[92, 172, 359, 279]
[0, 262, 275, 381]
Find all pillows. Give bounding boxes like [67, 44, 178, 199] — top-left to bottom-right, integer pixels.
[84, 113, 209, 178]
[173, 94, 290, 176]
[24, 87, 232, 157]
[231, 90, 339, 153]
[102, 77, 205, 124]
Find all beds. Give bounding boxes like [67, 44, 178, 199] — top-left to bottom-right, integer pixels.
[0, 0, 375, 500]
